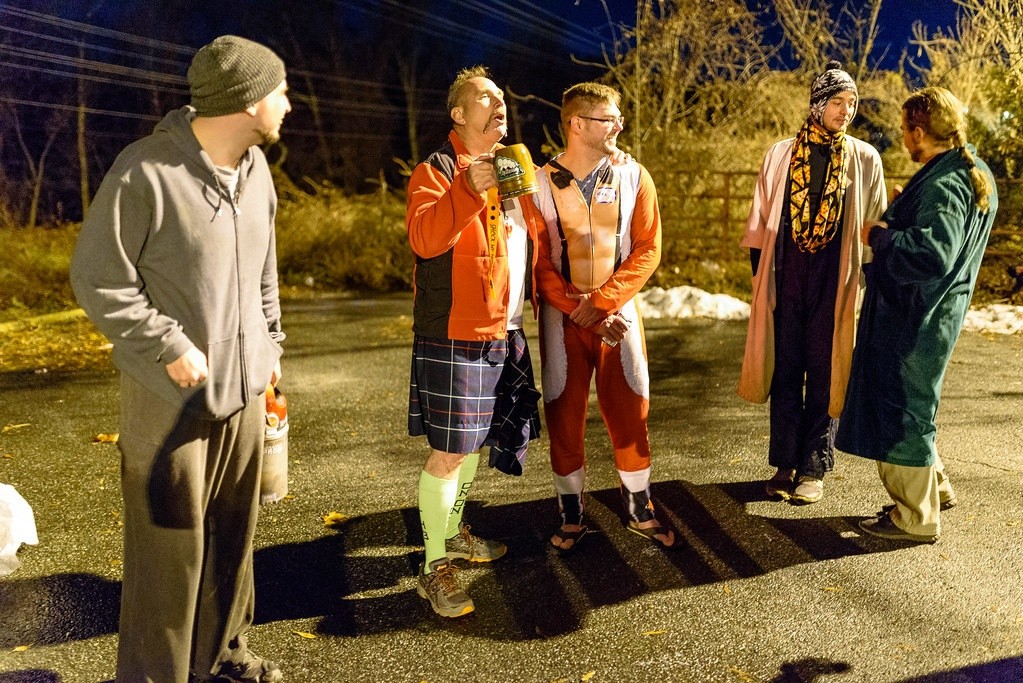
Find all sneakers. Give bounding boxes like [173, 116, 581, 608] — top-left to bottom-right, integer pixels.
[195, 648, 283, 683]
[445, 521, 507, 563]
[416, 557, 475, 618]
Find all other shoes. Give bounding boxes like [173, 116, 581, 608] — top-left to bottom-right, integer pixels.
[882, 496, 957, 512]
[790, 476, 823, 505]
[859, 513, 940, 543]
[765, 470, 795, 502]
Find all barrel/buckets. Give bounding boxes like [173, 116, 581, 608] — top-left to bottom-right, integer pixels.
[259, 422, 289, 507]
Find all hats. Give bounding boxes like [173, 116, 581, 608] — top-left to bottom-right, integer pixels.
[809, 59, 859, 126]
[187, 35, 286, 117]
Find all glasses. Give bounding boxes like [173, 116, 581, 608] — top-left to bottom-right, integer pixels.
[569, 114, 624, 127]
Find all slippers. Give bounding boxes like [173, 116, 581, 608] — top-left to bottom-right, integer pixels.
[626, 524, 683, 550]
[549, 524, 588, 553]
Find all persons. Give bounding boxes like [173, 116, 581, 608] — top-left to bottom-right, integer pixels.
[737, 60, 888, 504]
[407, 61, 543, 617]
[536, 82, 682, 557]
[70, 35, 293, 683]
[833, 87, 998, 543]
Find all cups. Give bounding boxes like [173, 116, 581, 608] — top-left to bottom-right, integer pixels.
[479, 143, 540, 202]
[602, 313, 632, 348]
